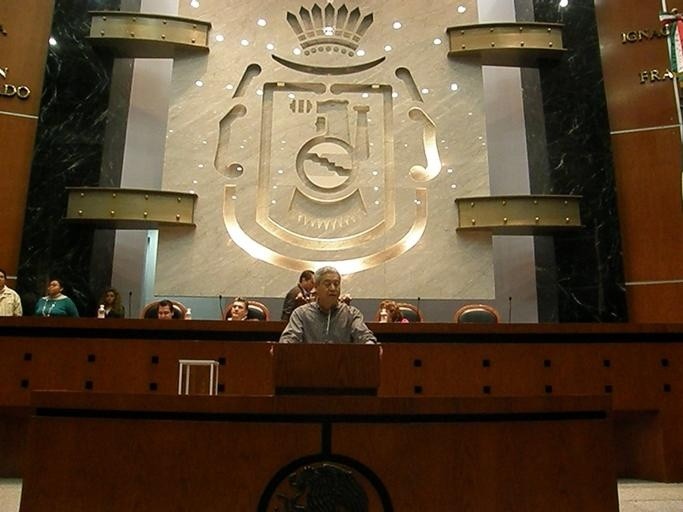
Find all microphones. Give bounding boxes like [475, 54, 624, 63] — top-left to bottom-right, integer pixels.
[129, 291, 132, 319]
[219, 295, 224, 320]
[416, 297, 420, 321]
[508, 296, 512, 323]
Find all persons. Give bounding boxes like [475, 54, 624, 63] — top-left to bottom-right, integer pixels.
[268, 265, 384, 360]
[281, 270, 315, 320]
[373, 298, 409, 323]
[155, 299, 175, 320]
[32, 276, 79, 318]
[0, 266, 23, 317]
[96, 286, 124, 320]
[225, 295, 260, 321]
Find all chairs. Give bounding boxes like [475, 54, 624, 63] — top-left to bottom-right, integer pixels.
[455, 304, 501, 325]
[224, 301, 269, 322]
[140, 301, 186, 319]
[398, 303, 424, 322]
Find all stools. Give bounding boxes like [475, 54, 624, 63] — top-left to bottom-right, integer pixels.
[178, 359, 220, 396]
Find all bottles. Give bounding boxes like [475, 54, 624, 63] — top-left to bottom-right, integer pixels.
[184, 307, 192, 321]
[97, 304, 105, 318]
[379, 308, 389, 323]
